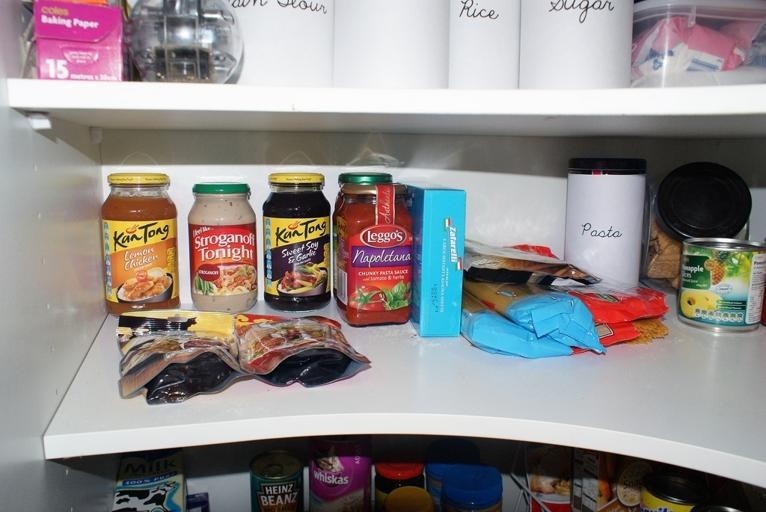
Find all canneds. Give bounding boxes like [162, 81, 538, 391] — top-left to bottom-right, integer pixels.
[187, 182, 258, 312]
[262, 173, 331, 313]
[639, 474, 697, 512]
[249, 450, 304, 512]
[676, 237, 766, 333]
[337, 183, 414, 327]
[333, 172, 392, 300]
[374, 451, 425, 512]
[100, 172, 180, 316]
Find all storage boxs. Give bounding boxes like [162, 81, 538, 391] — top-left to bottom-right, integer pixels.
[631, 2, 766, 89]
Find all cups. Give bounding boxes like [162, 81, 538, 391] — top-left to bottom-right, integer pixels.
[563, 157, 646, 288]
[521, 2, 632, 88]
[228, 2, 334, 88]
[336, 0, 449, 88]
[447, 0, 522, 87]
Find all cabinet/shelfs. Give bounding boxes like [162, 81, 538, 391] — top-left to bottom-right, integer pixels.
[0, 2, 766, 512]
